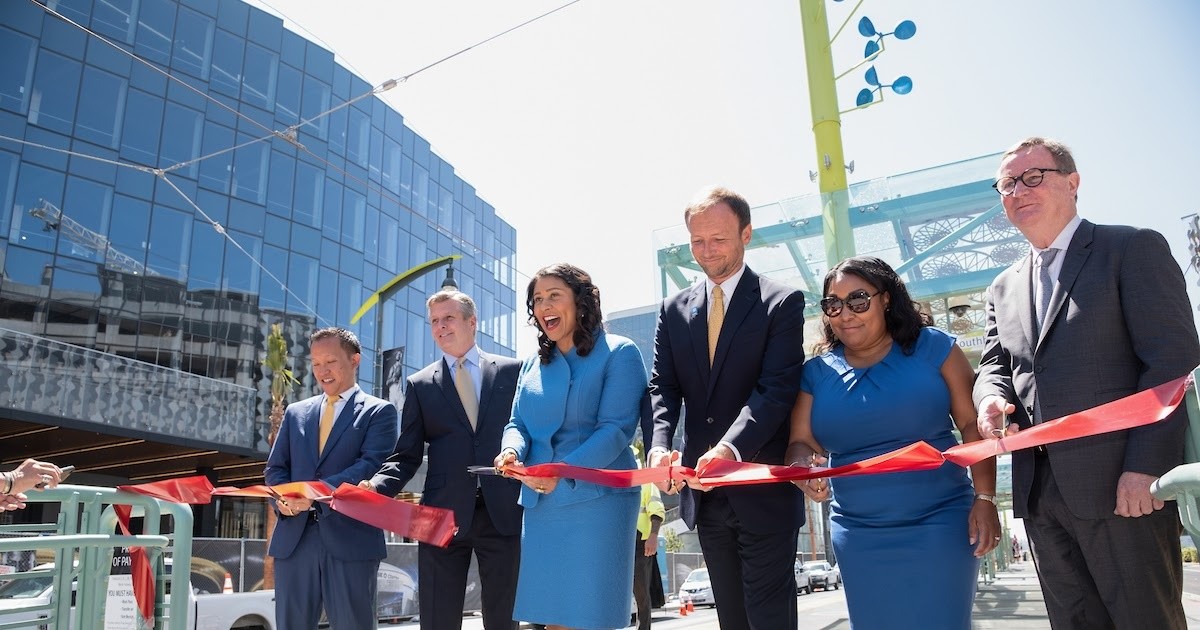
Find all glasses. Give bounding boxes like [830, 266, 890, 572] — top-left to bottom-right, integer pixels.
[820, 289, 880, 317]
[991, 167, 1058, 197]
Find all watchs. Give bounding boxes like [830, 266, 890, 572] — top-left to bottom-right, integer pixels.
[976, 494, 998, 506]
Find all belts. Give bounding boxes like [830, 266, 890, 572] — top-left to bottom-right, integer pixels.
[475, 488, 483, 503]
[308, 510, 319, 522]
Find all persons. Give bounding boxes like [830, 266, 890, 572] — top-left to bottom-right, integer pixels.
[628, 443, 666, 630]
[262, 329, 398, 630]
[0, 458, 64, 512]
[970, 137, 1200, 630]
[355, 291, 524, 630]
[639, 191, 808, 630]
[781, 257, 1005, 630]
[487, 265, 655, 630]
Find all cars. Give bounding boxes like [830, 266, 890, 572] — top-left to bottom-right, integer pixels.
[803, 562, 839, 591]
[0, 556, 196, 630]
[794, 558, 812, 594]
[678, 567, 716, 608]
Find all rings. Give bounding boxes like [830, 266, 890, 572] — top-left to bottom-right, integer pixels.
[994, 536, 1001, 541]
[817, 486, 822, 493]
[534, 485, 545, 494]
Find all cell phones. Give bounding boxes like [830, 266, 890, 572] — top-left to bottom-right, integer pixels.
[36, 465, 75, 488]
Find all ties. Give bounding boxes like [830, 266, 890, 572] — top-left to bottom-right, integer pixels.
[707, 286, 723, 370]
[319, 395, 340, 453]
[1035, 249, 1059, 338]
[456, 358, 478, 432]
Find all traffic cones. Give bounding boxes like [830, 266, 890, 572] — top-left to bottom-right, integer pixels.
[687, 595, 695, 612]
[680, 596, 687, 615]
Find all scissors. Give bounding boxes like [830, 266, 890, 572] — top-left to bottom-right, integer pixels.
[267, 486, 310, 516]
[313, 478, 371, 501]
[990, 399, 1010, 458]
[467, 450, 517, 477]
[804, 451, 822, 492]
[665, 450, 682, 494]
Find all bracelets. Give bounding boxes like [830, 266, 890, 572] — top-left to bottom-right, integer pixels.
[1, 472, 14, 495]
